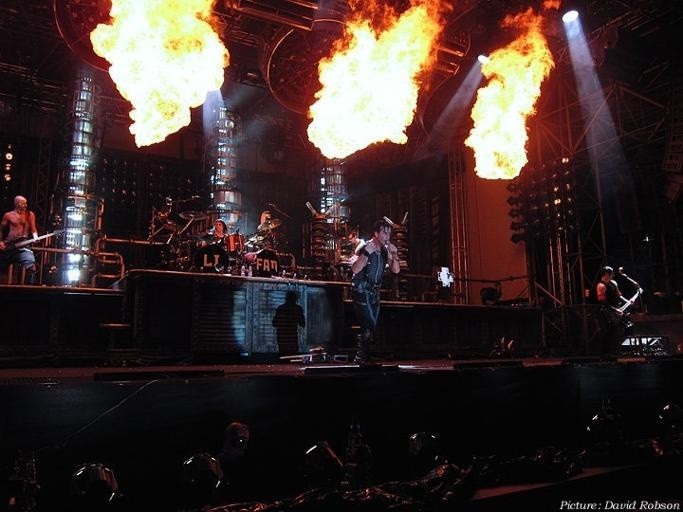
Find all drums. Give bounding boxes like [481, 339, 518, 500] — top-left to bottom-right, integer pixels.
[251, 249, 279, 277]
[195, 245, 229, 273]
[223, 234, 245, 252]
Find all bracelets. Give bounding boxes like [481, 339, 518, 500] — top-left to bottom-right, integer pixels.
[362, 249, 368, 256]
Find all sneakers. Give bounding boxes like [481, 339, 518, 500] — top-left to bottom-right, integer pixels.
[354, 351, 370, 366]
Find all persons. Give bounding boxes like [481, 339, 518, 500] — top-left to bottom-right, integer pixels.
[1, 195, 39, 285]
[350, 219, 401, 364]
[256, 210, 283, 245]
[593, 265, 639, 362]
[195, 219, 229, 274]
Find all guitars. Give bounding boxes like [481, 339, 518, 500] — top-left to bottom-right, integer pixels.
[0, 230, 64, 273]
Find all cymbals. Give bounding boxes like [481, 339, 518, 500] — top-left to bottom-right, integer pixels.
[179, 211, 209, 221]
[259, 218, 282, 230]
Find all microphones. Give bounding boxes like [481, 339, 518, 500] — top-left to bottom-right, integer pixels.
[306, 200, 316, 216]
[384, 239, 401, 264]
